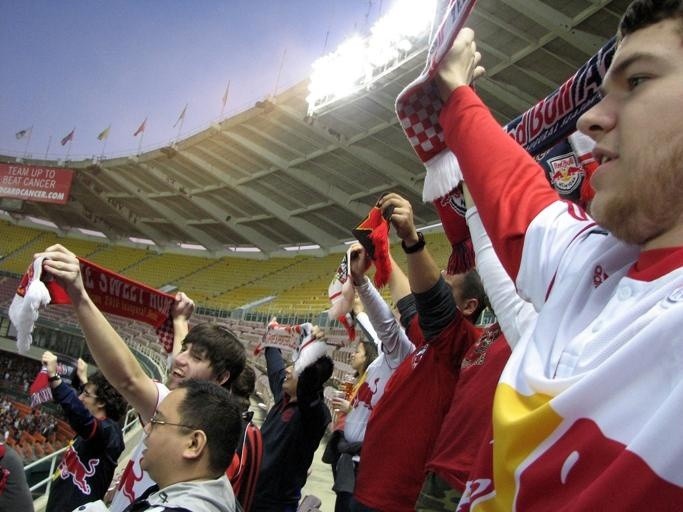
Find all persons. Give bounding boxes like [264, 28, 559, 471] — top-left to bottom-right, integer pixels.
[433, 1, 683, 512]
[1, 239, 337, 512]
[318, 157, 541, 512]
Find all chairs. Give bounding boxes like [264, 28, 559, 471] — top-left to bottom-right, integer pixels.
[298, 495, 321, 512]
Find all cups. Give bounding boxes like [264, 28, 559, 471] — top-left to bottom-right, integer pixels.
[344, 381, 354, 397]
[333, 391, 345, 413]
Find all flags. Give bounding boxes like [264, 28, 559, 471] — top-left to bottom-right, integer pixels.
[95, 126, 110, 142]
[132, 118, 147, 137]
[222, 80, 231, 106]
[172, 106, 188, 130]
[15, 127, 33, 141]
[60, 127, 75, 146]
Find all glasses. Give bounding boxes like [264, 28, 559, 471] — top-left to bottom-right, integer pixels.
[149, 416, 196, 429]
